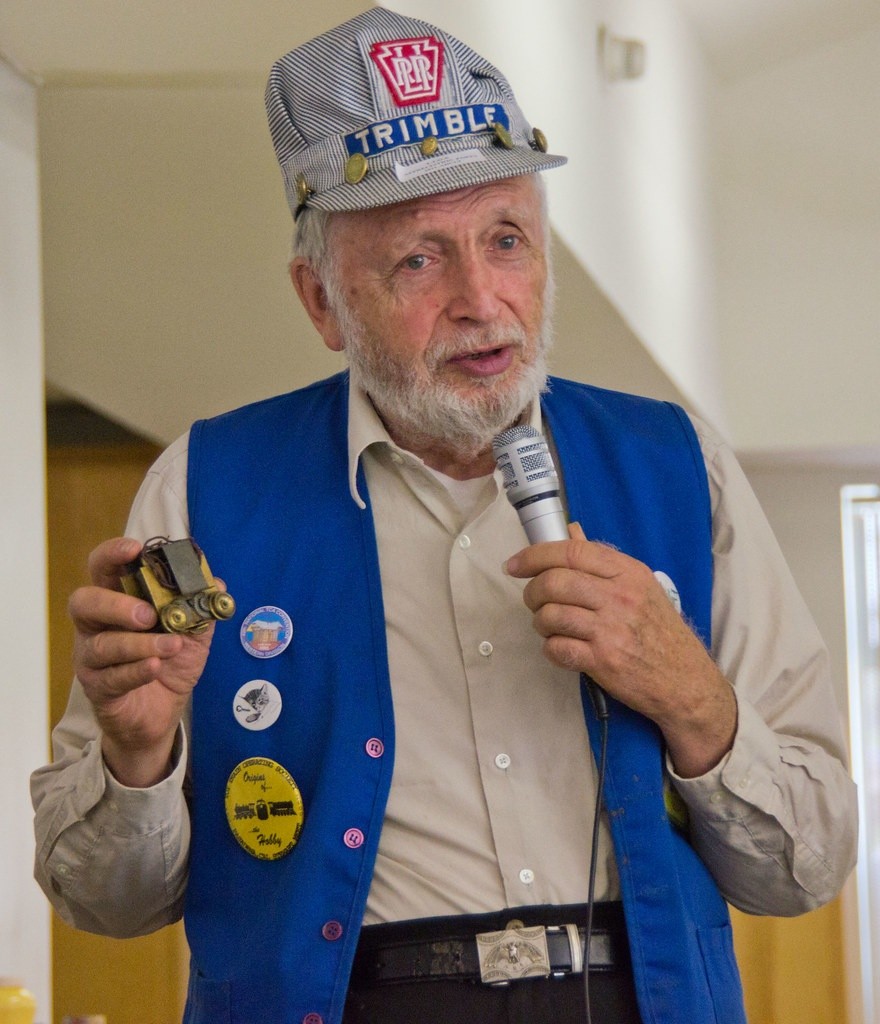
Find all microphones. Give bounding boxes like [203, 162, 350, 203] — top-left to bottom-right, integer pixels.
[493, 424, 609, 720]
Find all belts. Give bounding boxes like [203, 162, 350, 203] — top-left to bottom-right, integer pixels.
[350, 924, 628, 989]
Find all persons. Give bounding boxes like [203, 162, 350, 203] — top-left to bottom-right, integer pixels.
[26, 6, 860, 1023]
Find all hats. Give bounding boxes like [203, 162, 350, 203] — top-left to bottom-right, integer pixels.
[264, 5, 567, 224]
